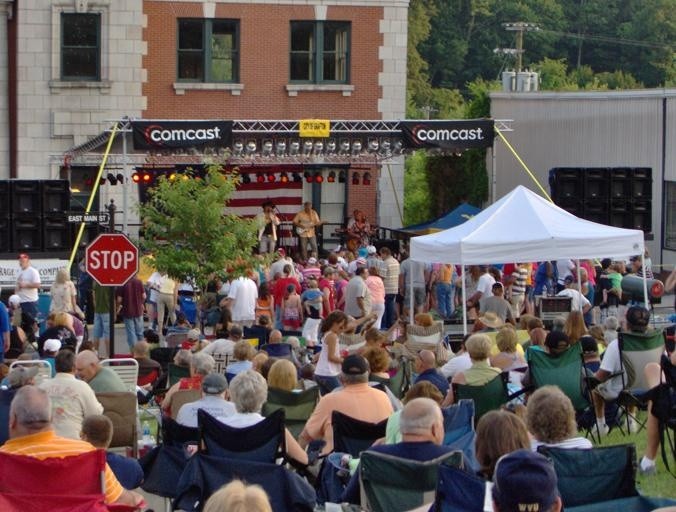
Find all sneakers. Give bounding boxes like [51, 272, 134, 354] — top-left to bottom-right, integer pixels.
[637, 460, 657, 476]
[590, 425, 610, 438]
[625, 418, 638, 431]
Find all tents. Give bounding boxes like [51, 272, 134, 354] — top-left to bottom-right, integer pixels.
[409, 185, 645, 268]
[391, 201, 481, 235]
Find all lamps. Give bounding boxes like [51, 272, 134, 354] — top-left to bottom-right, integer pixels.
[327, 171, 336, 183]
[99, 177, 105, 185]
[242, 173, 250, 184]
[256, 172, 265, 183]
[314, 172, 323, 183]
[267, 173, 276, 182]
[338, 171, 346, 182]
[363, 172, 371, 185]
[304, 172, 314, 183]
[132, 173, 141, 182]
[83, 174, 93, 185]
[280, 172, 288, 182]
[117, 173, 128, 184]
[108, 173, 117, 185]
[292, 172, 301, 182]
[142, 173, 151, 182]
[352, 171, 359, 185]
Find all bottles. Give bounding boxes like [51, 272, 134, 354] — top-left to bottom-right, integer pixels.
[142, 420, 151, 445]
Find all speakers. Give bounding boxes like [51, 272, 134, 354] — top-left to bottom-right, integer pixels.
[11, 179, 41, 259]
[42, 179, 71, 259]
[0, 179, 10, 259]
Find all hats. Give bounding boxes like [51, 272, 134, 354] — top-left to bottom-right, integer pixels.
[495, 450, 557, 511]
[44, 339, 61, 351]
[202, 374, 227, 393]
[8, 365, 38, 383]
[367, 246, 376, 254]
[324, 267, 337, 274]
[543, 332, 570, 349]
[479, 311, 502, 328]
[342, 354, 368, 373]
[627, 306, 650, 326]
[187, 328, 201, 341]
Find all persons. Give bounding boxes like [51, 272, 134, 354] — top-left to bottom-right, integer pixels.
[489, 447, 563, 512]
[41, 340, 62, 376]
[215, 319, 229, 339]
[333, 273, 348, 312]
[298, 274, 323, 348]
[231, 324, 243, 342]
[299, 355, 394, 475]
[77, 257, 93, 315]
[79, 415, 143, 490]
[150, 268, 168, 328]
[276, 265, 302, 313]
[1, 367, 37, 443]
[139, 215, 157, 253]
[412, 313, 432, 327]
[301, 281, 324, 319]
[552, 316, 567, 330]
[90, 279, 116, 355]
[319, 265, 338, 317]
[590, 326, 608, 354]
[603, 314, 617, 346]
[251, 352, 266, 371]
[522, 327, 547, 386]
[523, 319, 543, 350]
[261, 358, 277, 375]
[199, 338, 209, 353]
[286, 336, 307, 364]
[6, 294, 39, 355]
[122, 341, 162, 390]
[596, 259, 620, 310]
[136, 248, 156, 328]
[246, 314, 272, 348]
[215, 308, 240, 336]
[346, 266, 372, 335]
[292, 202, 320, 260]
[255, 281, 274, 325]
[372, 380, 444, 444]
[176, 373, 234, 432]
[255, 202, 280, 253]
[490, 328, 526, 406]
[644, 247, 653, 266]
[202, 479, 273, 512]
[366, 266, 386, 328]
[314, 310, 379, 402]
[174, 329, 201, 368]
[565, 309, 600, 371]
[201, 280, 232, 327]
[268, 273, 281, 292]
[473, 282, 517, 330]
[520, 385, 594, 454]
[474, 410, 532, 482]
[629, 256, 655, 278]
[165, 313, 192, 334]
[279, 284, 303, 330]
[268, 359, 304, 394]
[148, 333, 160, 344]
[161, 353, 217, 416]
[559, 276, 593, 314]
[593, 306, 650, 433]
[600, 262, 626, 307]
[43, 270, 85, 320]
[352, 211, 370, 248]
[519, 313, 532, 326]
[270, 246, 291, 278]
[379, 247, 401, 326]
[457, 259, 576, 311]
[158, 270, 180, 335]
[362, 245, 383, 269]
[440, 334, 491, 376]
[580, 259, 597, 289]
[353, 328, 384, 354]
[345, 398, 474, 512]
[341, 242, 381, 275]
[413, 350, 449, 400]
[216, 370, 309, 467]
[637, 340, 676, 473]
[262, 329, 301, 368]
[477, 310, 500, 333]
[15, 254, 41, 349]
[0, 298, 11, 365]
[568, 267, 596, 331]
[293, 251, 339, 278]
[224, 341, 253, 380]
[435, 263, 457, 320]
[348, 210, 370, 249]
[118, 277, 147, 348]
[133, 329, 160, 358]
[221, 267, 258, 328]
[75, 350, 128, 391]
[197, 328, 243, 374]
[42, 350, 105, 440]
[0, 385, 144, 512]
[44, 311, 83, 353]
[520, 331, 593, 415]
[364, 348, 393, 391]
[439, 333, 504, 406]
[399, 255, 431, 321]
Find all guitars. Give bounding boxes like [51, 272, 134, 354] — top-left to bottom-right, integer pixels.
[295, 219, 332, 234]
[346, 223, 382, 251]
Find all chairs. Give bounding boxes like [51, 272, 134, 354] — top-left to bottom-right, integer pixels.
[197, 408, 286, 466]
[528, 341, 601, 446]
[537, 442, 641, 509]
[157, 390, 204, 445]
[209, 353, 237, 375]
[10, 360, 52, 382]
[0, 448, 146, 512]
[607, 329, 668, 436]
[359, 451, 462, 512]
[441, 399, 478, 469]
[98, 358, 138, 411]
[437, 461, 484, 511]
[94, 392, 137, 459]
[260, 343, 292, 360]
[168, 362, 190, 389]
[331, 411, 389, 456]
[452, 371, 509, 421]
[110, 354, 157, 386]
[161, 414, 201, 512]
[261, 385, 320, 441]
